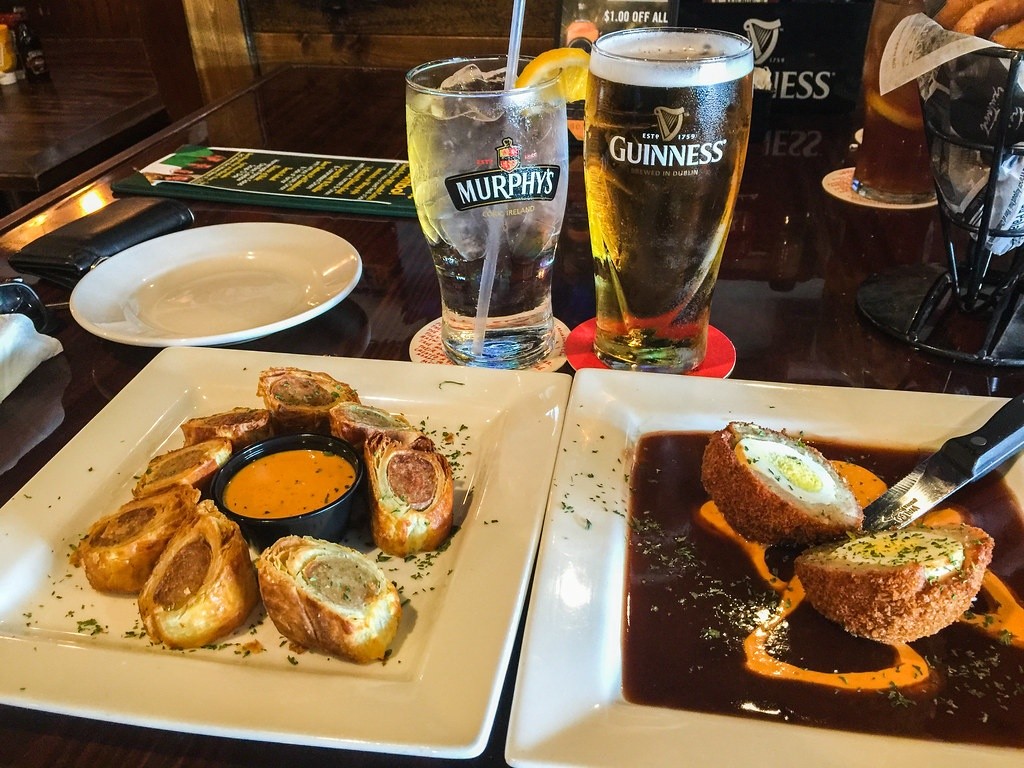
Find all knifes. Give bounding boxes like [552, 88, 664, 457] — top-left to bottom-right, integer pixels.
[857, 381, 1024, 535]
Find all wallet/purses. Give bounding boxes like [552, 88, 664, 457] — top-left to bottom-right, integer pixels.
[9, 196, 197, 288]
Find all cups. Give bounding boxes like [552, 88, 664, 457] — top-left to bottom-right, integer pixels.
[584, 24, 753, 374]
[406, 55, 561, 368]
[850, 0, 946, 201]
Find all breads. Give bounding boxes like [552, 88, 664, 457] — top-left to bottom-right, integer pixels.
[87, 359, 453, 673]
[701, 412, 999, 656]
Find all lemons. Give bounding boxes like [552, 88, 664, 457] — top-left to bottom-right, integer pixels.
[511, 49, 595, 117]
[860, 76, 934, 132]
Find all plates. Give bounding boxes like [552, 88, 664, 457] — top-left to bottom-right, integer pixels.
[0, 345, 570, 758]
[504, 370, 1024, 766]
[69, 221, 364, 352]
